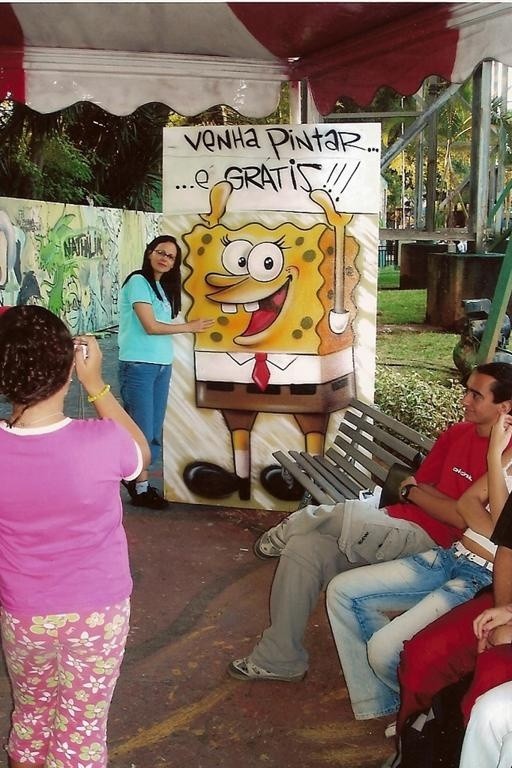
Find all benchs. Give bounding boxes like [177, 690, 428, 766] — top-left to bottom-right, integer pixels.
[271, 397, 437, 511]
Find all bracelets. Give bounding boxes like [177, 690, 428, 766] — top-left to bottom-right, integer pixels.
[83, 384, 116, 400]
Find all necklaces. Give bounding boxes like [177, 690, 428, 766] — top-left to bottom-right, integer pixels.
[9, 411, 66, 428]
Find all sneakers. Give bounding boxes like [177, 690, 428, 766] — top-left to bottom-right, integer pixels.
[121, 478, 170, 510]
[253, 516, 288, 561]
[227, 655, 306, 683]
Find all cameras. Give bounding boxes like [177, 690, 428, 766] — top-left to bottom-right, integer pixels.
[71, 342, 89, 367]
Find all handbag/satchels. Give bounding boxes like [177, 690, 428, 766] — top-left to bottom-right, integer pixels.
[397, 709, 464, 768]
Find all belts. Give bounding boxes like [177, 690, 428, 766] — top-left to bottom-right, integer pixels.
[454, 541, 493, 573]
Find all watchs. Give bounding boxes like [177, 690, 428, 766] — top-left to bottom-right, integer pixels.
[400, 483, 419, 502]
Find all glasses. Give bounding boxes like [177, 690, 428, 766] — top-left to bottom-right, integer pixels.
[151, 248, 175, 261]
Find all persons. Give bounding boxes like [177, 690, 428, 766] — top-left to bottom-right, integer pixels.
[377, 482, 511, 744]
[455, 680, 512, 768]
[113, 233, 216, 511]
[2, 304, 155, 766]
[322, 407, 511, 742]
[232, 362, 511, 681]
